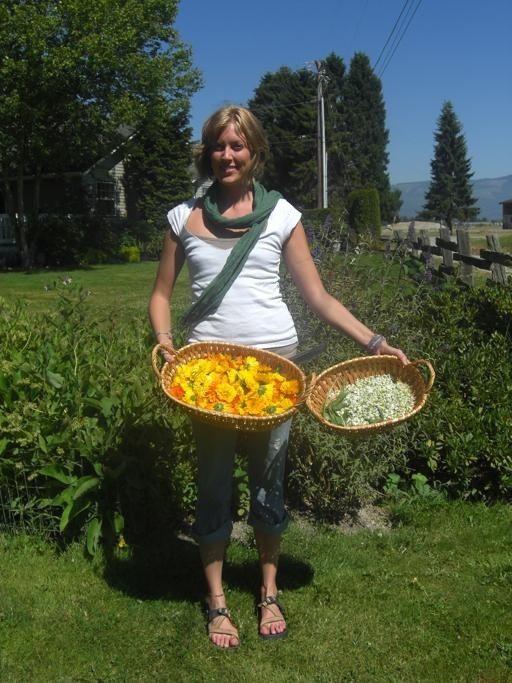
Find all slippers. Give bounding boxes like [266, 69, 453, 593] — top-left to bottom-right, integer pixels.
[207, 608, 241, 651]
[257, 595, 287, 639]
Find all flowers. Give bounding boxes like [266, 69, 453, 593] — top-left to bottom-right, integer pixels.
[168, 349, 301, 418]
[320, 372, 418, 428]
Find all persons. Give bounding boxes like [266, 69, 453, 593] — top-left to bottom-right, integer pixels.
[146, 105, 413, 649]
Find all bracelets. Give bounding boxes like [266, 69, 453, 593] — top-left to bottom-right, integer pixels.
[155, 332, 173, 339]
[367, 334, 386, 354]
